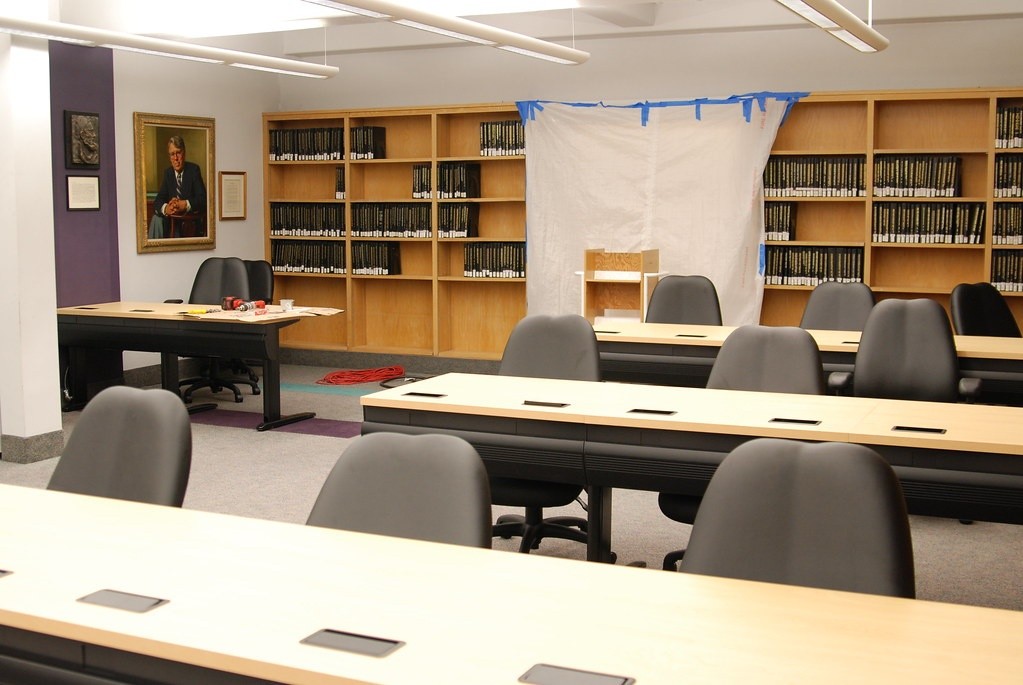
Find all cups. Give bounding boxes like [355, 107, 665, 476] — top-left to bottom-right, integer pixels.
[278, 299, 294, 312]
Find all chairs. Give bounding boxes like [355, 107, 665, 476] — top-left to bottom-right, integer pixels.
[490, 314, 618, 563]
[828, 298, 982, 527]
[801, 281, 875, 332]
[658, 326, 827, 573]
[46, 384, 193, 507]
[646, 275, 723, 326]
[306, 431, 492, 549]
[177, 257, 275, 403]
[950, 282, 1022, 338]
[677, 438, 916, 599]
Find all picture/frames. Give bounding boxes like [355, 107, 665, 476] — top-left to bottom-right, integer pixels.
[218, 170, 247, 221]
[66, 174, 101, 211]
[133, 112, 217, 255]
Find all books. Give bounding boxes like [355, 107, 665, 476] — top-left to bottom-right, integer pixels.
[270, 202, 480, 238]
[480, 119, 525, 157]
[994, 152, 1023, 198]
[763, 202, 798, 240]
[995, 107, 1023, 148]
[763, 155, 963, 197]
[463, 243, 526, 278]
[764, 245, 864, 286]
[269, 125, 386, 161]
[991, 248, 1023, 292]
[413, 161, 481, 199]
[873, 200, 986, 244]
[992, 201, 1023, 245]
[271, 242, 400, 276]
[335, 166, 346, 200]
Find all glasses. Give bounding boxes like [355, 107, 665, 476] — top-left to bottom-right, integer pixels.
[168, 149, 183, 158]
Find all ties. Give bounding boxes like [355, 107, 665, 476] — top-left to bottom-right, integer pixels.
[176, 172, 182, 199]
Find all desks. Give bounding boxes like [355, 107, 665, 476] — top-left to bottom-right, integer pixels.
[359, 371, 1023, 564]
[0, 482, 1023, 685]
[56, 301, 345, 432]
[592, 326, 1023, 408]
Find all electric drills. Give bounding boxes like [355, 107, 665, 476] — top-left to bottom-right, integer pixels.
[221, 297, 265, 312]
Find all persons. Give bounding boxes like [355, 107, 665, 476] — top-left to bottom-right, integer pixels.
[148, 135, 208, 239]
[72, 115, 100, 164]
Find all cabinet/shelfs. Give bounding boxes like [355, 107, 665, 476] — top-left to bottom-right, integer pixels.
[262, 86, 1023, 362]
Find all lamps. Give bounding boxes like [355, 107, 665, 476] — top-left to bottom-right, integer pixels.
[301, 0, 590, 67]
[772, 0, 889, 55]
[0, 16, 340, 80]
